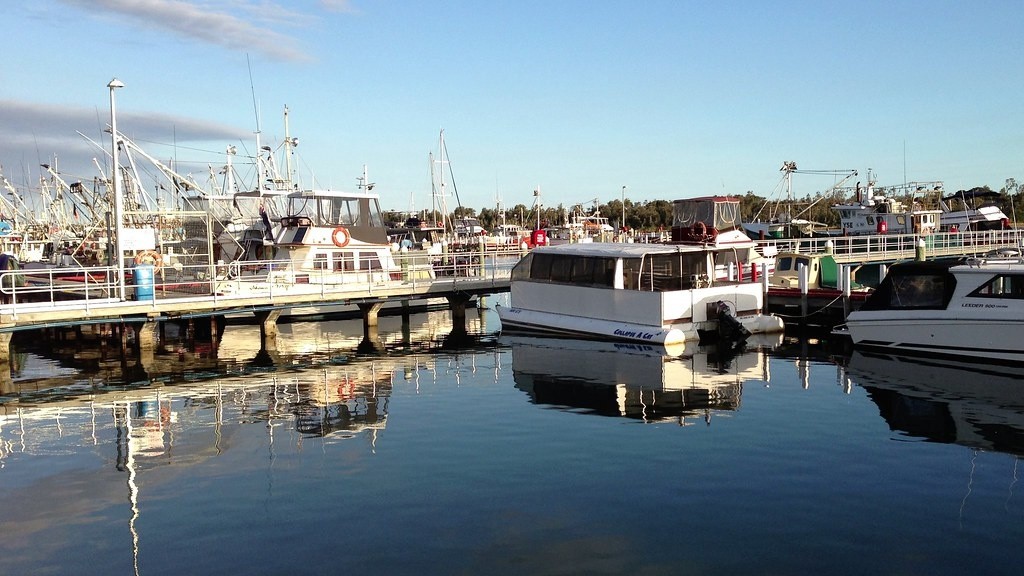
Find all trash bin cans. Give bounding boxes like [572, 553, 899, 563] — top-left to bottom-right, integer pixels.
[130, 263, 153, 300]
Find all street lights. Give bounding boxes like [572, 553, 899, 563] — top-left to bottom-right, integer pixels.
[103, 78, 127, 303]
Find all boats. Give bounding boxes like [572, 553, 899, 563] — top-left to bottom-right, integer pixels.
[1, 62, 1024, 331]
[830, 229, 1024, 362]
[494, 218, 786, 359]
[495, 330, 774, 429]
[838, 347, 1024, 461]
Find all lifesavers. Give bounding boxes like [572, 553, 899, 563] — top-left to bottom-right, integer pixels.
[135, 249, 162, 275]
[438, 222, 443, 227]
[691, 221, 707, 239]
[332, 226, 350, 247]
